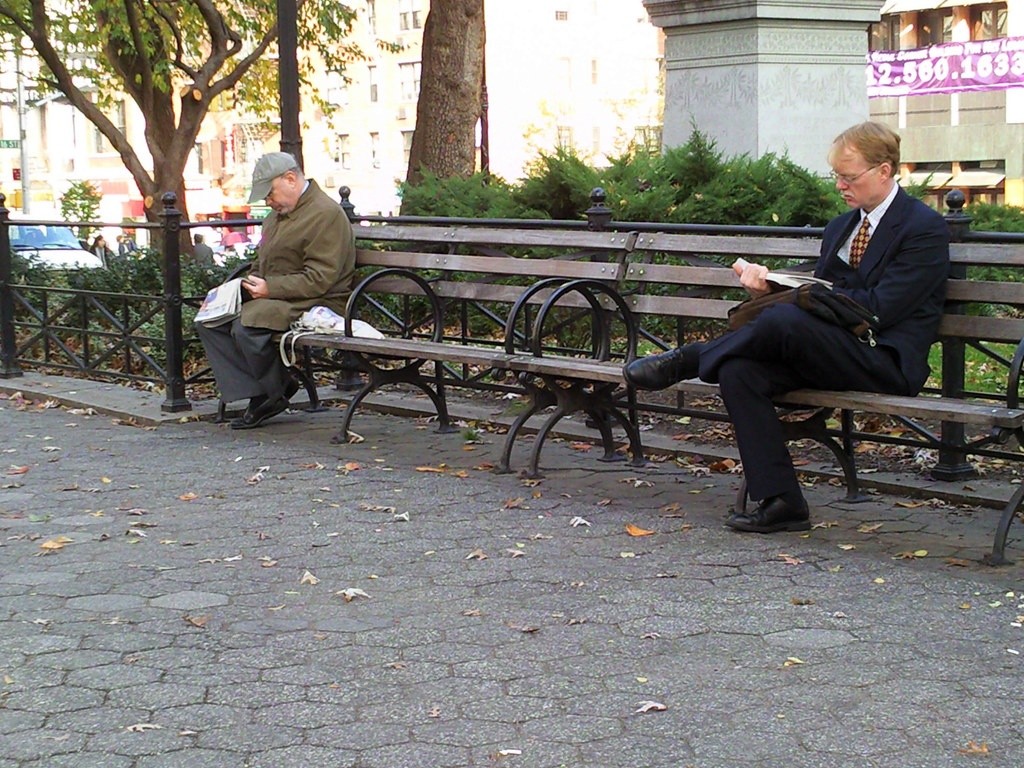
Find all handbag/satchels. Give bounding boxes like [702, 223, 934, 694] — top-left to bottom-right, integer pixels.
[727, 283, 882, 347]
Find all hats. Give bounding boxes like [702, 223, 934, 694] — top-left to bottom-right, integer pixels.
[247, 152, 298, 204]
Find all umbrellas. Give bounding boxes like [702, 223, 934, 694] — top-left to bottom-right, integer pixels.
[220, 232, 251, 247]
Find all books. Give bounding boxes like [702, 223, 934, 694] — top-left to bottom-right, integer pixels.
[732, 256, 833, 290]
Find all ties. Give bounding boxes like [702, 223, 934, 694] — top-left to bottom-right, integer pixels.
[850, 212, 874, 270]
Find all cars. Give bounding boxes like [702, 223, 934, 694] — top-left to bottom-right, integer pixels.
[8, 222, 104, 270]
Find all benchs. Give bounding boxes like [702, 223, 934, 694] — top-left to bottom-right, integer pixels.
[519, 234, 1022, 567]
[213, 224, 639, 474]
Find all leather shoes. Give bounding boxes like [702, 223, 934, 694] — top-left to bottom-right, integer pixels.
[622, 347, 685, 392]
[231, 377, 299, 429]
[726, 497, 811, 533]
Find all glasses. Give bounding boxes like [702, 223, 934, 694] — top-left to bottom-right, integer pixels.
[829, 165, 883, 182]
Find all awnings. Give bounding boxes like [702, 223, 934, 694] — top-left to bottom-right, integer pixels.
[897, 168, 1005, 190]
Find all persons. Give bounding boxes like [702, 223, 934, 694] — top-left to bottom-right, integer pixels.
[622, 120, 950, 533]
[190, 234, 216, 266]
[86, 235, 138, 268]
[197, 151, 356, 430]
